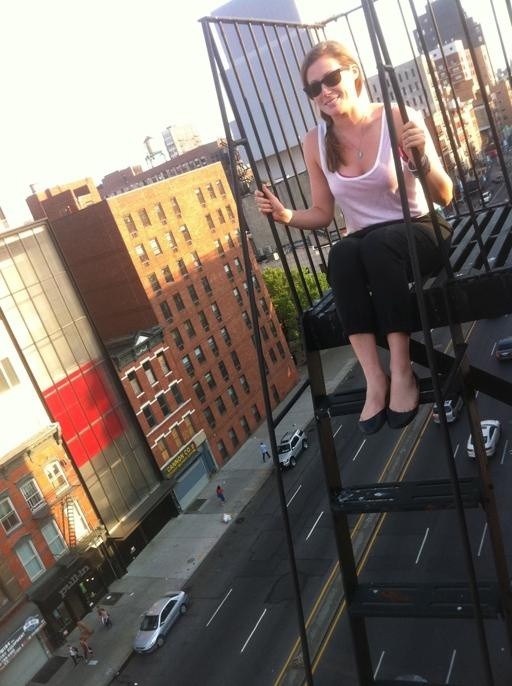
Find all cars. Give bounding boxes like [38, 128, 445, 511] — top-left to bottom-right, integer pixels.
[276, 429, 308, 468]
[132, 590, 191, 655]
[466, 419, 501, 459]
[479, 191, 492, 205]
[496, 338, 512, 361]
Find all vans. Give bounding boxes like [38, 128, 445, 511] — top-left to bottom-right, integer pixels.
[432, 394, 464, 424]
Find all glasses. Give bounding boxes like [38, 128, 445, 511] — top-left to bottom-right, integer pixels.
[303, 67, 348, 97]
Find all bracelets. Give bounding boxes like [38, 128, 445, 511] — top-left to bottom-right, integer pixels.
[407, 155, 431, 178]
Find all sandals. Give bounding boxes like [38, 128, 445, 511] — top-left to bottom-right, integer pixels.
[358, 371, 420, 434]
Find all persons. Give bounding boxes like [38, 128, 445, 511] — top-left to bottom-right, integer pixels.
[67, 606, 112, 667]
[260, 442, 270, 463]
[255, 41, 454, 436]
[216, 485, 225, 501]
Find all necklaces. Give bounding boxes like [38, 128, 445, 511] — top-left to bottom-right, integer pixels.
[334, 113, 366, 159]
